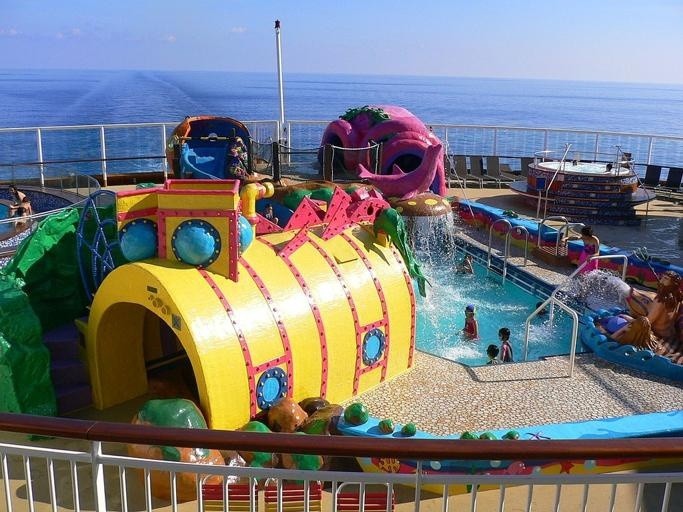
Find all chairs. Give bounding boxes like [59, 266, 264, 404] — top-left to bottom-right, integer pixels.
[446, 154, 515, 189]
[655, 167, 683, 197]
[638, 165, 663, 194]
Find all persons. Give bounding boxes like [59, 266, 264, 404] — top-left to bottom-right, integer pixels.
[497, 327, 515, 362]
[578, 226, 600, 275]
[573, 159, 578, 167]
[454, 304, 478, 339]
[459, 254, 475, 276]
[484, 344, 503, 364]
[261, 204, 280, 226]
[609, 314, 662, 353]
[14, 206, 30, 232]
[535, 300, 548, 316]
[7, 185, 27, 219]
[602, 164, 612, 174]
[618, 270, 681, 338]
[13, 196, 35, 226]
